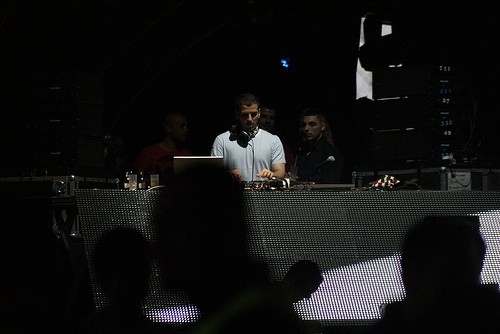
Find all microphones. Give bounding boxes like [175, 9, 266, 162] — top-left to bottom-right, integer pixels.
[312, 156, 335, 170]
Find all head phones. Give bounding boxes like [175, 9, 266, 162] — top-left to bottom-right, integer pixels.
[232, 125, 259, 141]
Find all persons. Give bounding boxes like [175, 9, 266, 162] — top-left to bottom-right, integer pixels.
[0, 94, 500, 334]
[210, 94, 286, 183]
[367, 214, 500, 334]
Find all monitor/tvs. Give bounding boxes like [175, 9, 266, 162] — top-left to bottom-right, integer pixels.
[173, 156, 224, 174]
